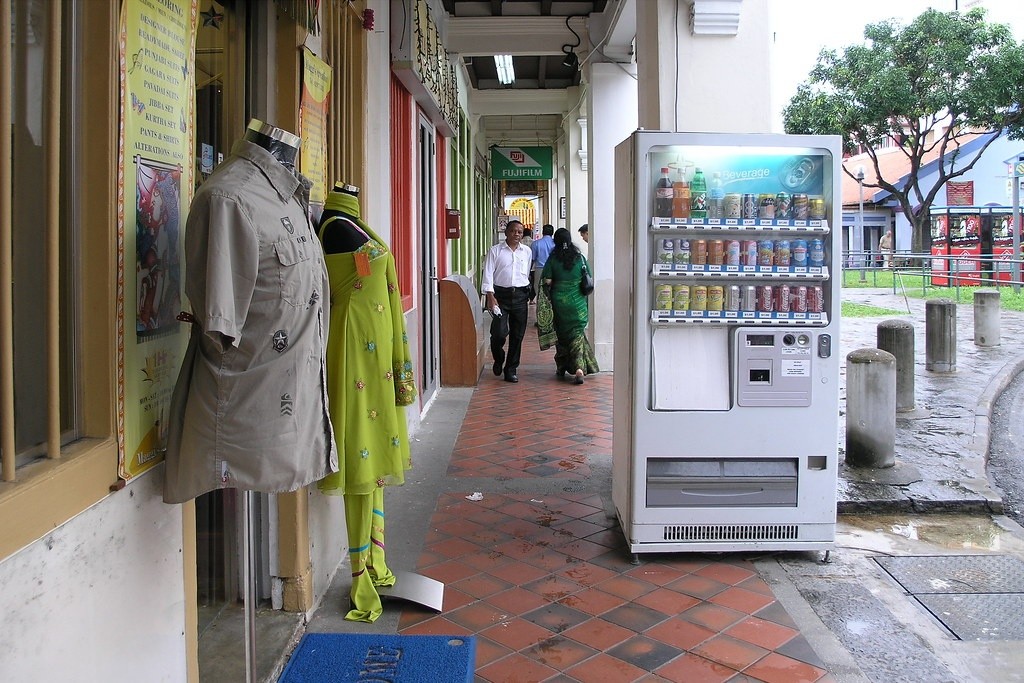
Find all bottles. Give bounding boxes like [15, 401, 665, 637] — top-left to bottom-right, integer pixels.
[673, 168, 690, 218]
[655, 168, 673, 217]
[690, 166, 707, 218]
[709, 172, 724, 219]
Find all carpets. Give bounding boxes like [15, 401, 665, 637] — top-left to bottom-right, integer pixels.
[277, 633, 476, 683]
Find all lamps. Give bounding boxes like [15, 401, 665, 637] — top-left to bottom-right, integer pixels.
[562, 14, 581, 68]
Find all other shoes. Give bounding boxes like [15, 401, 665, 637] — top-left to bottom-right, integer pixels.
[529, 300, 536, 305]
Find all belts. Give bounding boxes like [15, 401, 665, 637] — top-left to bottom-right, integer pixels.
[494, 285, 530, 292]
[882, 246, 890, 249]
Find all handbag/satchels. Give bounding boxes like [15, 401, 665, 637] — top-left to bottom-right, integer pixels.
[580, 254, 594, 295]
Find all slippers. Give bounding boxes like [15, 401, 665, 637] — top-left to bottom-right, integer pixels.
[557, 369, 565, 376]
[574, 377, 584, 385]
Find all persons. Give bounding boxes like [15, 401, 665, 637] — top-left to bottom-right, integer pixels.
[162, 118, 338, 503]
[530, 224, 555, 326]
[579, 224, 589, 243]
[936, 219, 945, 236]
[879, 230, 891, 270]
[519, 228, 534, 303]
[482, 219, 533, 382]
[320, 180, 415, 623]
[998, 213, 1023, 238]
[956, 215, 978, 237]
[536, 227, 600, 382]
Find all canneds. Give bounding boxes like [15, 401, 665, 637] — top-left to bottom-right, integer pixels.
[656, 238, 825, 267]
[723, 192, 826, 221]
[655, 283, 824, 312]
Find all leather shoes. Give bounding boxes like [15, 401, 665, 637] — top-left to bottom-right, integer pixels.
[493, 357, 505, 376]
[504, 372, 518, 382]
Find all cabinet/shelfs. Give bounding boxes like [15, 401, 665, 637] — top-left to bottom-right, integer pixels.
[440, 274, 485, 387]
